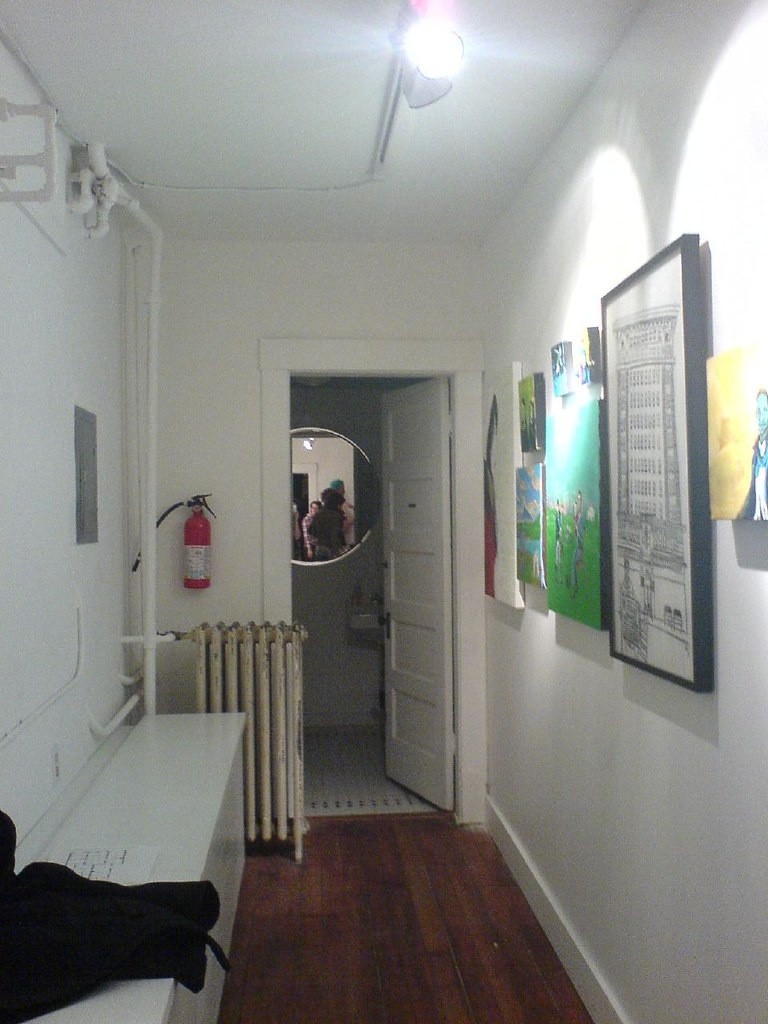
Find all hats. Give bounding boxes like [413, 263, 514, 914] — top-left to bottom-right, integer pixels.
[330, 479, 343, 489]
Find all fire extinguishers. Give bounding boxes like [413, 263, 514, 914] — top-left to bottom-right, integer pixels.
[179, 493, 216, 589]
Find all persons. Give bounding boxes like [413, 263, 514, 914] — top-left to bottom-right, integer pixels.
[292, 480, 354, 561]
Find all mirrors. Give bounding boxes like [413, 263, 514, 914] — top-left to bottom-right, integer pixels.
[289, 427, 380, 566]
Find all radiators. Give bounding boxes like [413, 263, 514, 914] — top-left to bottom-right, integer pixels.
[192, 622, 308, 866]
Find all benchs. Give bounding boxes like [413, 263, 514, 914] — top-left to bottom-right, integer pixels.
[0, 712, 248, 1024]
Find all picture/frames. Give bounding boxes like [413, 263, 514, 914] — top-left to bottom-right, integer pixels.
[600, 234, 714, 694]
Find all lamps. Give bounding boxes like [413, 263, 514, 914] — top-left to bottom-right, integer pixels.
[399, 17, 466, 111]
[302, 437, 316, 452]
